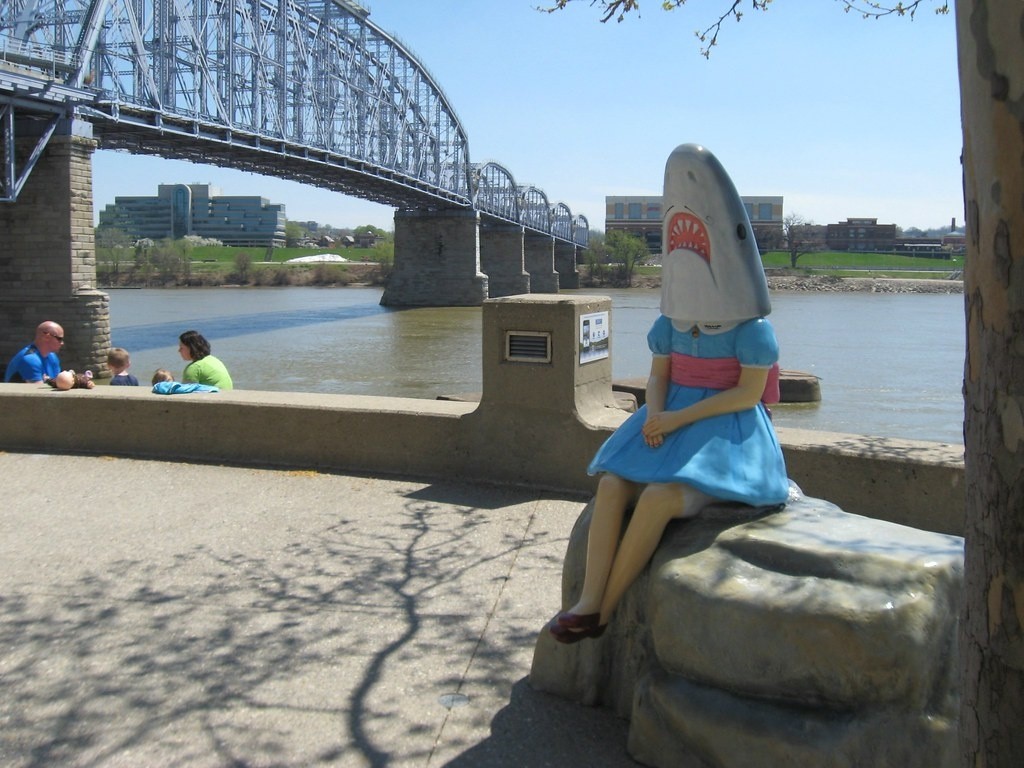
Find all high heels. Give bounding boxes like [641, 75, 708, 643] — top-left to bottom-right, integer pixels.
[558, 612, 601, 629]
[550, 623, 608, 644]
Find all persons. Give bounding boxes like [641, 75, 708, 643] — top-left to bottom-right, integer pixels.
[178, 331, 233, 390]
[5, 321, 65, 384]
[108, 348, 139, 386]
[43, 368, 95, 390]
[547, 143, 789, 646]
[152, 368, 174, 387]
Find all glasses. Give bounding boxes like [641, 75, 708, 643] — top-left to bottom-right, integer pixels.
[43, 331, 64, 342]
